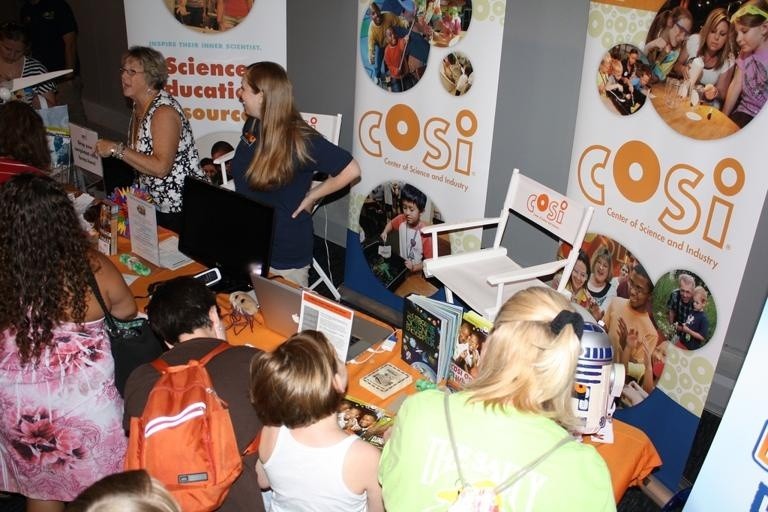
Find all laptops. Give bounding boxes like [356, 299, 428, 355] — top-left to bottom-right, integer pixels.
[248, 272, 391, 364]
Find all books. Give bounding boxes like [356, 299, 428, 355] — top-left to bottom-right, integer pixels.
[96, 198, 119, 257]
[402, 293, 463, 386]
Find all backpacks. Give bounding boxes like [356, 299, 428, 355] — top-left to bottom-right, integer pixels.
[123, 342, 262, 512]
[439, 391, 582, 512]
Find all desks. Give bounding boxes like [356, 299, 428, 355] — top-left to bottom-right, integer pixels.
[650, 83, 740, 141]
[600, 84, 647, 117]
[59, 181, 662, 508]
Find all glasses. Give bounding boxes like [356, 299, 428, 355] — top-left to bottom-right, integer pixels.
[120, 67, 146, 76]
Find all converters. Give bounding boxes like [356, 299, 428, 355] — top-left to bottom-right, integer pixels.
[382, 336, 397, 352]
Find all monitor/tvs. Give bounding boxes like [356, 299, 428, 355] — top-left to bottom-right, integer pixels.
[178, 175, 277, 295]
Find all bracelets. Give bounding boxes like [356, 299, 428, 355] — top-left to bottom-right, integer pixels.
[110, 141, 127, 159]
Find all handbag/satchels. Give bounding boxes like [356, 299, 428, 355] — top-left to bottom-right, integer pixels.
[86, 267, 171, 398]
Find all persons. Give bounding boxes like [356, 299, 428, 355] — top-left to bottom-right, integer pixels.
[175, 0, 206, 28]
[722, 0, 767, 130]
[384, 25, 413, 92]
[248, 329, 384, 512]
[590, 264, 658, 371]
[564, 249, 591, 308]
[675, 286, 708, 350]
[412, 0, 452, 43]
[644, 7, 692, 84]
[611, 263, 629, 288]
[0, 24, 57, 109]
[1, 172, 136, 512]
[621, 328, 669, 393]
[28, 0, 87, 130]
[69, 468, 180, 512]
[338, 406, 362, 429]
[456, 323, 471, 346]
[442, 2, 461, 35]
[447, 52, 472, 95]
[352, 413, 376, 436]
[594, 47, 649, 106]
[666, 274, 694, 324]
[89, 46, 211, 234]
[617, 258, 652, 316]
[230, 62, 363, 289]
[456, 333, 482, 374]
[673, 7, 736, 109]
[215, 0, 253, 33]
[585, 245, 617, 311]
[375, 286, 618, 512]
[123, 276, 266, 512]
[381, 182, 433, 274]
[367, 0, 410, 84]
[199, 140, 234, 184]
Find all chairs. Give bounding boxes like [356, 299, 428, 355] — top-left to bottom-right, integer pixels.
[213, 112, 344, 301]
[420, 167, 595, 322]
